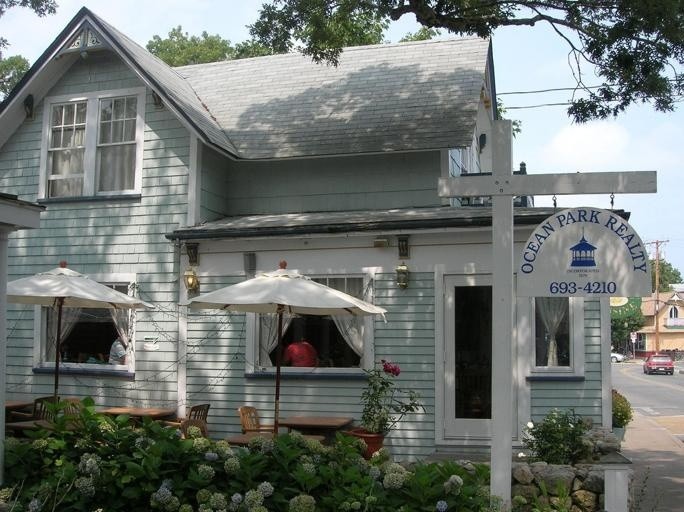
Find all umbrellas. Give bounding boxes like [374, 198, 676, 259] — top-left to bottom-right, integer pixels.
[4, 259, 157, 408]
[175, 259, 390, 433]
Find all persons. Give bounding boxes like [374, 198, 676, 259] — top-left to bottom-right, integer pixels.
[92, 352, 109, 361]
[279, 332, 322, 368]
[107, 333, 127, 366]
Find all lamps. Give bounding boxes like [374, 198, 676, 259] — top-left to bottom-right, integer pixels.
[396, 263, 408, 289]
[183, 266, 198, 291]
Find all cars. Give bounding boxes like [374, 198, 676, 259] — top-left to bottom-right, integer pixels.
[611, 345, 625, 363]
[643, 353, 674, 375]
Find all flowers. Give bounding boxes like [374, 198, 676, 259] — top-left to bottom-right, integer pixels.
[354, 357, 427, 436]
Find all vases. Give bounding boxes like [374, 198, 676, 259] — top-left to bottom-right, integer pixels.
[353, 431, 386, 461]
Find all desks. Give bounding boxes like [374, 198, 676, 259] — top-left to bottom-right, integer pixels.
[95, 405, 177, 426]
[225, 416, 355, 448]
[5, 401, 85, 433]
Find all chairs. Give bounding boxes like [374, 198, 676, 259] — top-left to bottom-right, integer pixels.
[238, 405, 274, 435]
[9, 396, 83, 424]
[155, 404, 210, 435]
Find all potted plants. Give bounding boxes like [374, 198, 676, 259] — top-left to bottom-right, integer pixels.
[611, 388, 633, 441]
